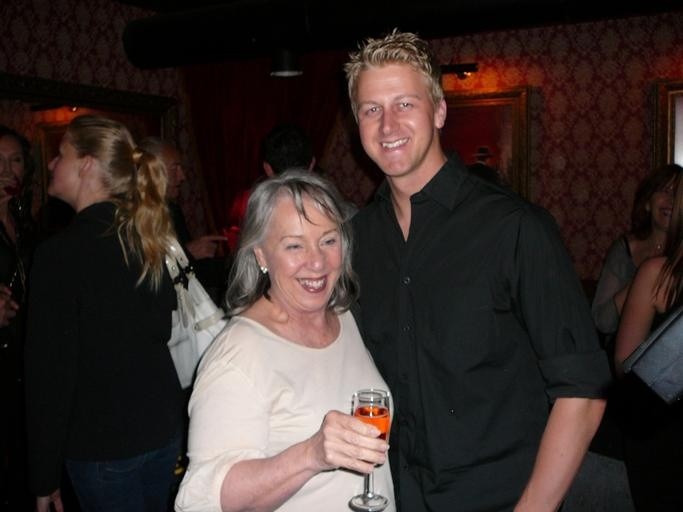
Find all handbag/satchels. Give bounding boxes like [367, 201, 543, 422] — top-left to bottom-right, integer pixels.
[164, 233, 229, 390]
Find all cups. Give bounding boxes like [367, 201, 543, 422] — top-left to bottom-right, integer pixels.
[348, 389, 392, 511]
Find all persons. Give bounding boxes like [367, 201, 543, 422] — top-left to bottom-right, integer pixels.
[139, 138, 230, 276]
[614, 173, 683, 512]
[19, 113, 185, 511]
[467, 147, 500, 187]
[0, 126, 30, 512]
[260, 127, 316, 179]
[591, 164, 683, 379]
[171, 169, 395, 512]
[342, 27, 632, 512]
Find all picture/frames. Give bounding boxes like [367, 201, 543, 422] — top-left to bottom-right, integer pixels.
[651, 76, 682, 171]
